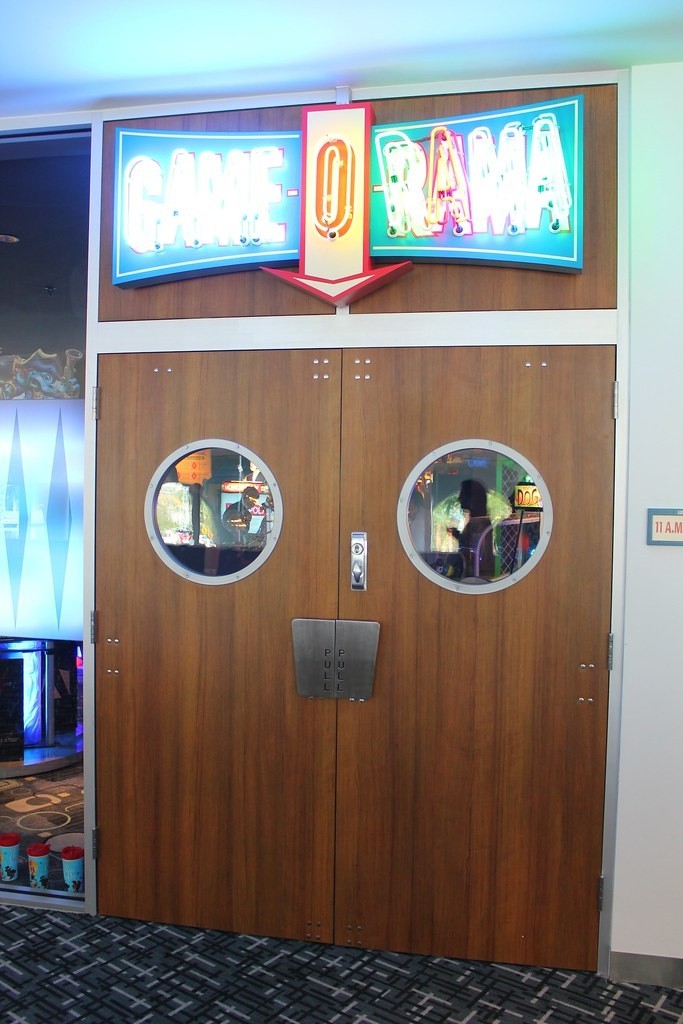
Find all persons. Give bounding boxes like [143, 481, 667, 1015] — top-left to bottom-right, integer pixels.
[220, 487, 258, 538]
[448, 480, 495, 576]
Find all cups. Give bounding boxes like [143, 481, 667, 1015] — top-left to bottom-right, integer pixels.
[26, 843, 50, 889]
[62, 845, 83, 894]
[0, 831, 20, 882]
